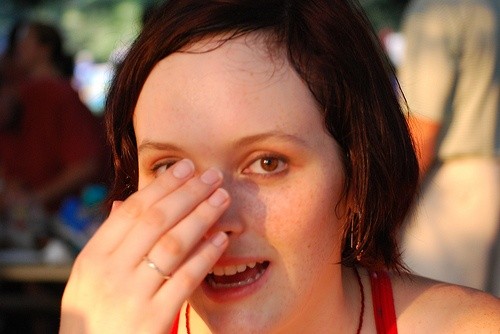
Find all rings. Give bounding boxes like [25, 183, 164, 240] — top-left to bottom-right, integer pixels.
[142, 253, 172, 284]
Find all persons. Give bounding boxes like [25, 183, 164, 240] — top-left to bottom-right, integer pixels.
[0, 1, 130, 272]
[390, 0, 500, 303]
[55, 1, 500, 334]
